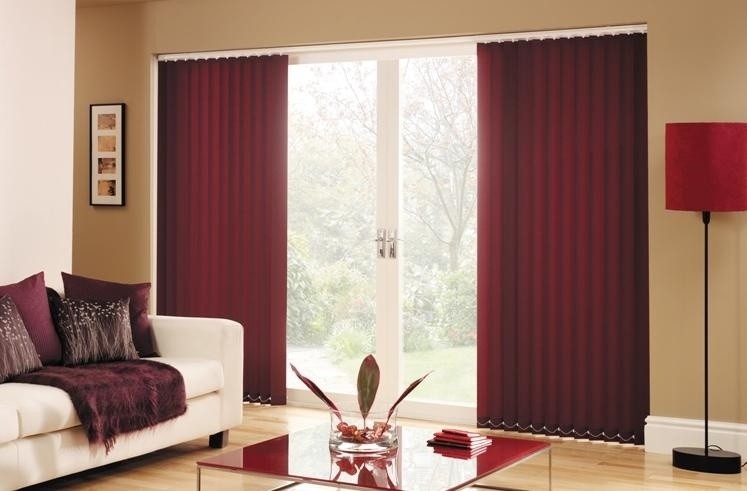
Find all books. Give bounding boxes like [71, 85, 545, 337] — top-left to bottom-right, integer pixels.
[426, 428, 493, 451]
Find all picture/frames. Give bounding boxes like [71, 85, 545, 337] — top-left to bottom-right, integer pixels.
[86, 101, 127, 206]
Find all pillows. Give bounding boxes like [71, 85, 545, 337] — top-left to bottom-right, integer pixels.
[0, 270, 158, 380]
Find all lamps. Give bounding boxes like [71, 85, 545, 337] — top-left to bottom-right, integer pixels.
[664, 119, 747, 472]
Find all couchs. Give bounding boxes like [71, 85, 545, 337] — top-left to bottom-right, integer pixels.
[0, 295, 246, 491]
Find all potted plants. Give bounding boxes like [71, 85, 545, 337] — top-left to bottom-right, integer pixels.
[291, 355, 435, 459]
